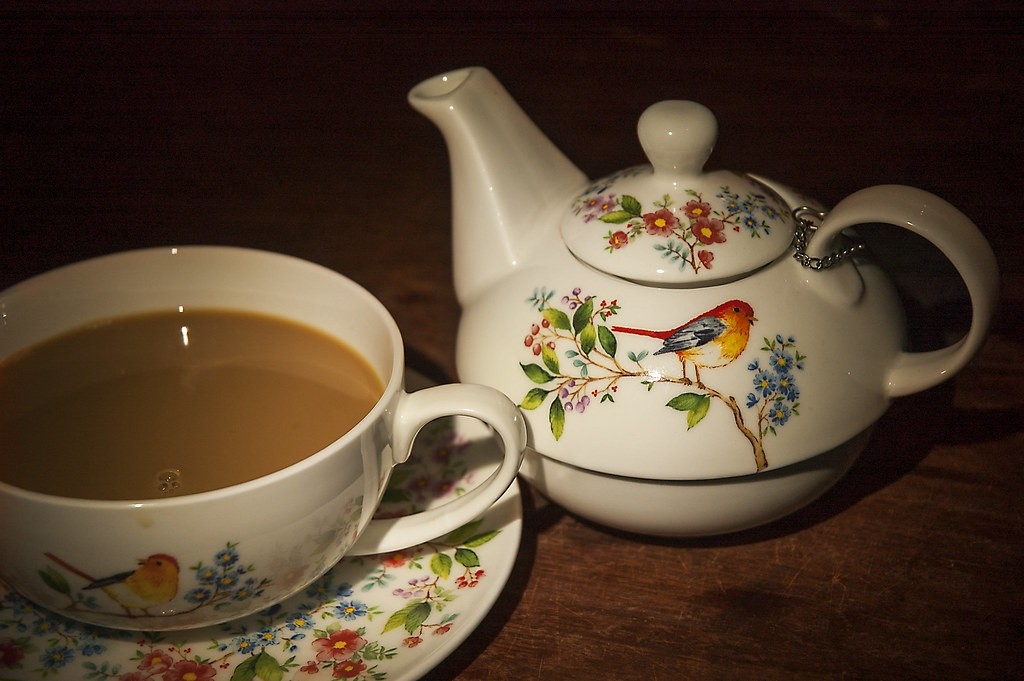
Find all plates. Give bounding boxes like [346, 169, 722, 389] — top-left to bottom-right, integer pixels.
[0, 370, 521, 681]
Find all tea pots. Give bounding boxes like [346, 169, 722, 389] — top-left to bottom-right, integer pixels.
[407, 68, 997, 537]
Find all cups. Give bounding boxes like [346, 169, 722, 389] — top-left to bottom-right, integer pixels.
[1, 247, 528, 630]
